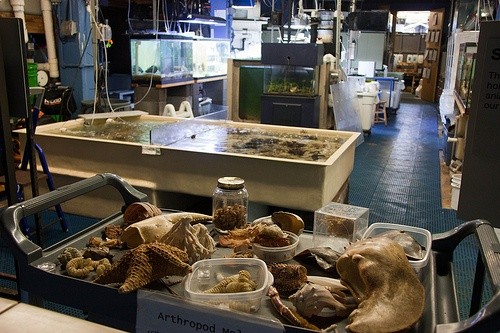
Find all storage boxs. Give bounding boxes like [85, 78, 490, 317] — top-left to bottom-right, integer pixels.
[349, 92, 377, 133]
[230, 20, 262, 60]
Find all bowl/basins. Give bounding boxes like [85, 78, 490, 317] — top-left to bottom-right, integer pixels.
[252, 216, 304, 236]
[250, 230, 300, 262]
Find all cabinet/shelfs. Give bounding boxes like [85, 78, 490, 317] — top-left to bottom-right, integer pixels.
[132, 74, 227, 120]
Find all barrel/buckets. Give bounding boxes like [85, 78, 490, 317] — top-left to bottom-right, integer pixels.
[451, 173, 462, 210]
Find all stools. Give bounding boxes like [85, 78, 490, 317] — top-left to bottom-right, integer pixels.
[371, 100, 388, 125]
